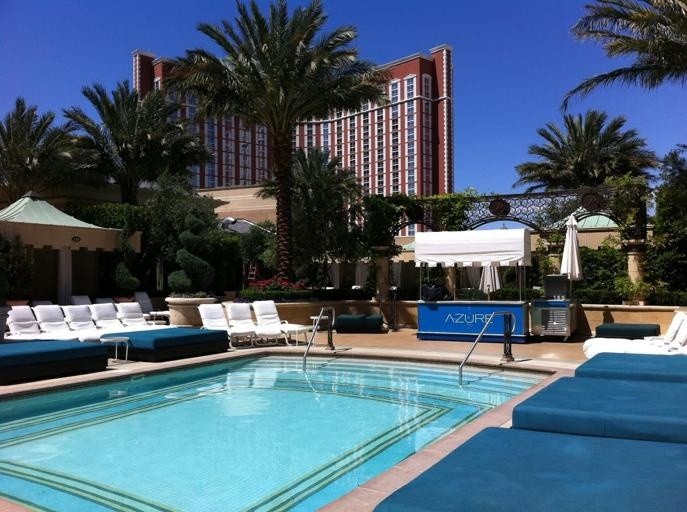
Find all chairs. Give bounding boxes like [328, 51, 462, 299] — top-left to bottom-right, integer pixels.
[511, 374, 686, 442]
[372, 427, 686, 510]
[7, 300, 181, 341]
[30, 291, 153, 314]
[196, 300, 320, 349]
[574, 349, 686, 381]
[582, 309, 687, 360]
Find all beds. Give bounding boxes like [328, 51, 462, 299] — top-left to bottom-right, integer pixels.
[0, 338, 112, 386]
[100, 326, 232, 365]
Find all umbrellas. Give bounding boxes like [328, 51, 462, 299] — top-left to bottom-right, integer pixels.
[479, 267, 501, 300]
[560, 213, 583, 298]
